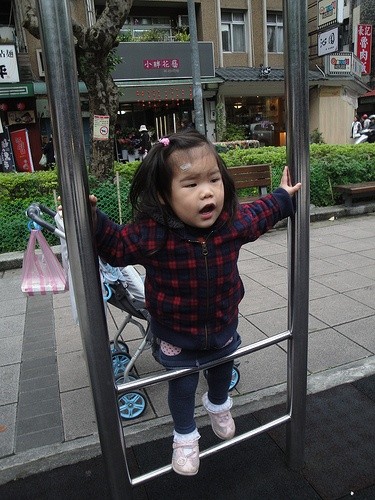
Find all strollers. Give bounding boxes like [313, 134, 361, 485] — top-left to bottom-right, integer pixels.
[27, 203, 241, 420]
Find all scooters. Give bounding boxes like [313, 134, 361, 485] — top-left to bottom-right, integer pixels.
[354, 126, 375, 148]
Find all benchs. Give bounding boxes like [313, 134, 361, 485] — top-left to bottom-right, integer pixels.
[226, 163, 276, 232]
[332, 181, 375, 207]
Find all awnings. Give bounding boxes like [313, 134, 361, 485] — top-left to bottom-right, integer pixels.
[216, 68, 328, 93]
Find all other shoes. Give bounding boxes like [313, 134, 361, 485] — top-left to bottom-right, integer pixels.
[201, 391, 236, 440]
[171, 427, 201, 476]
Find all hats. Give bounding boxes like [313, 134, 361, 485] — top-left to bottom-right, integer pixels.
[138, 125, 148, 132]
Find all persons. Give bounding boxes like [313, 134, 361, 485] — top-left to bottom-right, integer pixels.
[57, 131, 304, 475]
[43, 138, 56, 171]
[350, 114, 375, 144]
[135, 126, 150, 162]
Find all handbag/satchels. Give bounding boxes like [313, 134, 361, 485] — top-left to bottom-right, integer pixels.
[20, 229, 69, 298]
